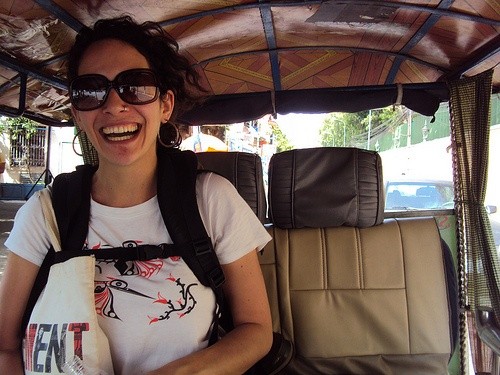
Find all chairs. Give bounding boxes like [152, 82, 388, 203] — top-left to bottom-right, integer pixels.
[415, 187, 435, 205]
[196, 147, 459, 375]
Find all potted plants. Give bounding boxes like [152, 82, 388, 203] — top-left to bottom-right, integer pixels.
[0, 152, 5, 173]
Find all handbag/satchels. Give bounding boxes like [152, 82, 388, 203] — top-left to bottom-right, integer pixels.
[208, 308, 322, 375]
[21, 187, 114, 375]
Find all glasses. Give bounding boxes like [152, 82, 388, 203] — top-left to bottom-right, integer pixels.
[68, 69, 160, 111]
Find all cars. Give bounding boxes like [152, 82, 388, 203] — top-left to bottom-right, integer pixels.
[384, 179, 499, 248]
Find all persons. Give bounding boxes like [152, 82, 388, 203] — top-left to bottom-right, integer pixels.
[0, 13, 274, 375]
